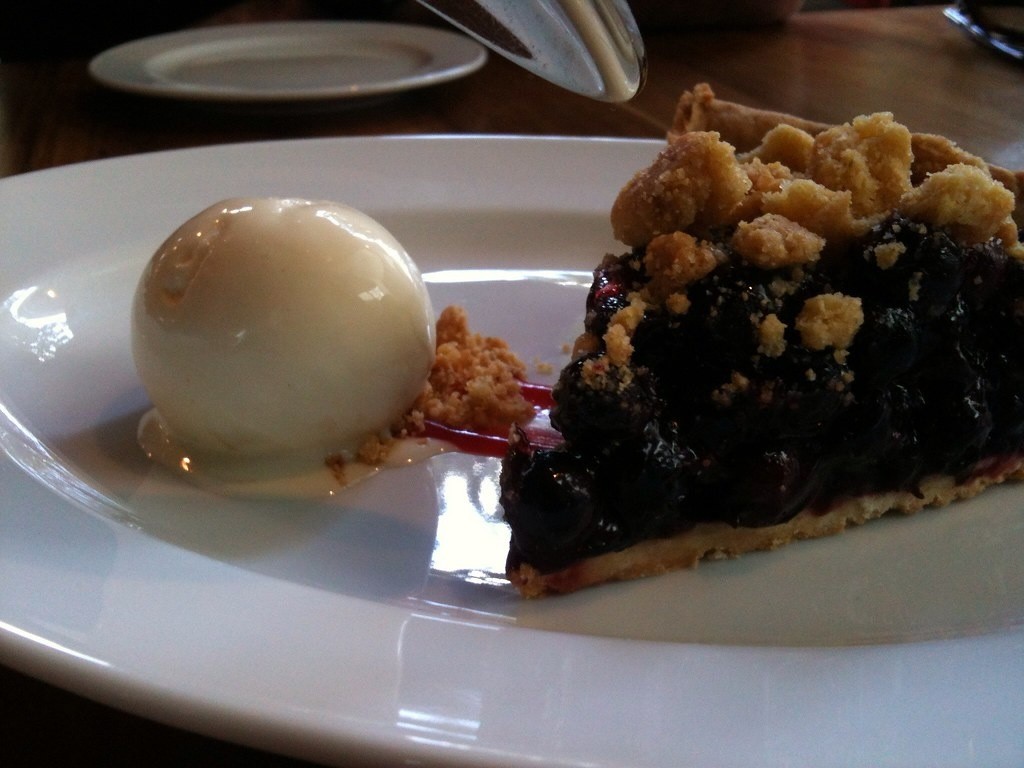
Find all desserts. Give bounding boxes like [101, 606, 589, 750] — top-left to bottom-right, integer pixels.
[130, 83, 1024, 594]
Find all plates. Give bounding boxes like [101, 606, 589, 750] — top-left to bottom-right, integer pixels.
[0, 131, 1024, 768]
[89, 21, 486, 101]
